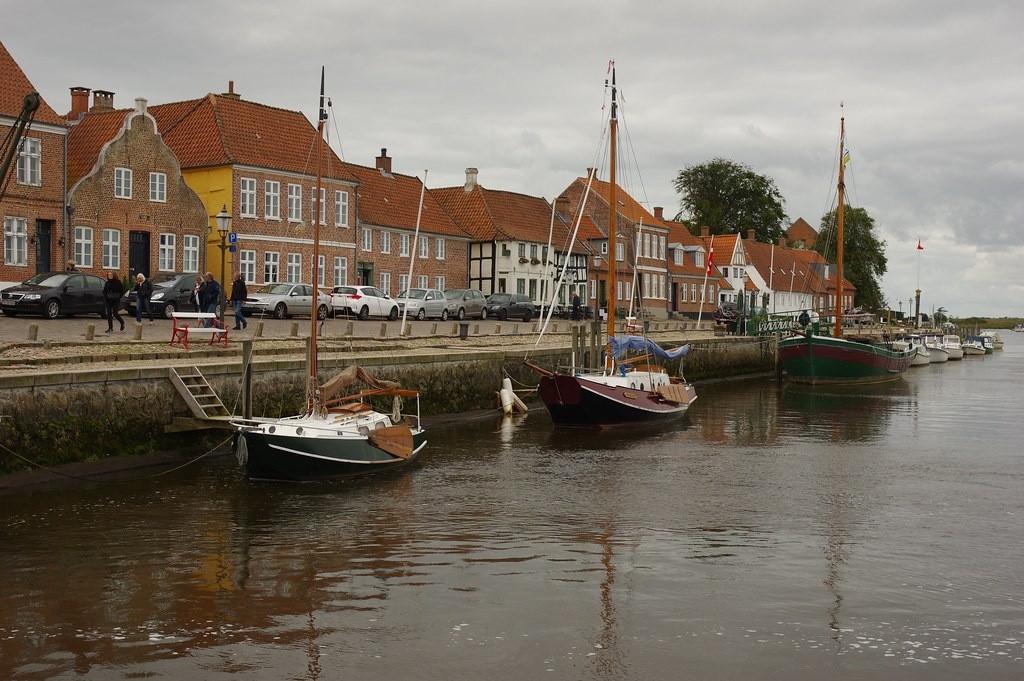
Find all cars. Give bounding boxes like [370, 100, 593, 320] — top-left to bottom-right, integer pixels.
[394, 288, 448, 321]
[241, 283, 332, 320]
[124, 272, 227, 320]
[329, 285, 400, 321]
[442, 289, 488, 320]
[0, 271, 125, 319]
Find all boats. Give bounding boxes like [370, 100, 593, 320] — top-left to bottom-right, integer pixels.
[941, 331, 964, 360]
[882, 330, 930, 367]
[1014, 323, 1024, 332]
[920, 324, 950, 363]
[961, 332, 1004, 355]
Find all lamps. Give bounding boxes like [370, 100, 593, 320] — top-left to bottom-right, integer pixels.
[29, 233, 37, 245]
[58, 237, 66, 245]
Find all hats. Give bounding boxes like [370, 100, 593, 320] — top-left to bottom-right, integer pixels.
[67, 260, 75, 266]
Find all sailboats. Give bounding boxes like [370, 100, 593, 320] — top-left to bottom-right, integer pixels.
[230, 65, 426, 481]
[523, 57, 699, 429]
[778, 98, 919, 386]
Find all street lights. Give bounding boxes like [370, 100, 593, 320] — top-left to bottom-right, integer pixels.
[909, 297, 913, 321]
[215, 204, 232, 337]
[742, 271, 749, 336]
[899, 300, 902, 312]
[592, 251, 602, 333]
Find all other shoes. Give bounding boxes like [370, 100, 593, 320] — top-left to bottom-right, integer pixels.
[233, 326, 241, 330]
[243, 319, 247, 329]
[105, 327, 115, 333]
[149, 321, 154, 325]
[120, 320, 125, 331]
[134, 321, 142, 326]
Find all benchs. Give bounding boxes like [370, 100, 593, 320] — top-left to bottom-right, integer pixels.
[624, 316, 644, 335]
[170, 310, 229, 351]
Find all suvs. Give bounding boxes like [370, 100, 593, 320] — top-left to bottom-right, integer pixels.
[485, 292, 535, 322]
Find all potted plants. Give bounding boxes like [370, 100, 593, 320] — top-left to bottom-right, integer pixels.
[519, 258, 553, 265]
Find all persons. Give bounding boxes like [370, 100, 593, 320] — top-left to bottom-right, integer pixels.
[133, 273, 154, 325]
[102, 270, 125, 333]
[187, 272, 221, 328]
[799, 310, 810, 333]
[67, 259, 78, 271]
[572, 292, 580, 321]
[230, 272, 247, 330]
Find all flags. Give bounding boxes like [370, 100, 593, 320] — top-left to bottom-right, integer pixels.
[843, 150, 850, 165]
